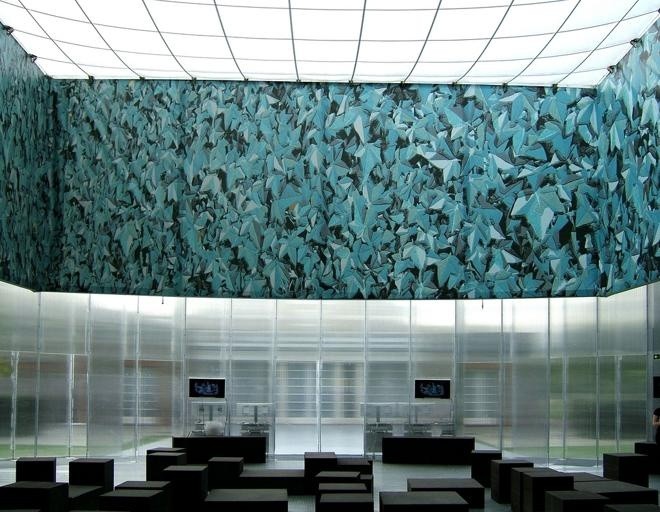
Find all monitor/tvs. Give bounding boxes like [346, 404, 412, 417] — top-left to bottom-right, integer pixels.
[188, 377, 226, 401]
[413, 379, 451, 398]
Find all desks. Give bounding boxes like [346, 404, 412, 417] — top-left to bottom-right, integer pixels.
[173, 430, 269, 464]
[381, 433, 475, 465]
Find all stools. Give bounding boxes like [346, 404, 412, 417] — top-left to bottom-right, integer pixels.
[0, 443, 660, 512]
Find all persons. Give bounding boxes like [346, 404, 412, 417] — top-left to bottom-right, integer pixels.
[653, 407, 660, 442]
